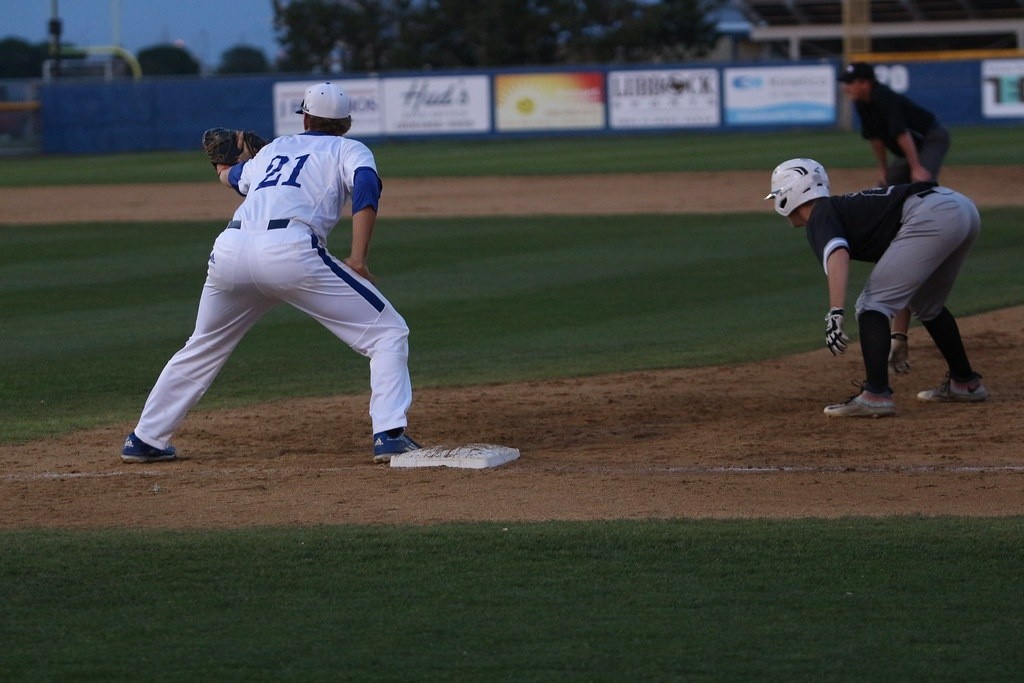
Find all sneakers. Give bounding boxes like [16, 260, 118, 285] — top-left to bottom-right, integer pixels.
[121, 432, 176, 463]
[373, 428, 423, 463]
[915, 371, 988, 404]
[823, 390, 896, 419]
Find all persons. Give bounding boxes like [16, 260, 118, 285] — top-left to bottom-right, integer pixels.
[120, 82, 427, 463]
[837, 61, 951, 187]
[765, 159, 990, 419]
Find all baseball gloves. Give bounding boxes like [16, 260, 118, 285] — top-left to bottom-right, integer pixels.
[200, 126, 269, 172]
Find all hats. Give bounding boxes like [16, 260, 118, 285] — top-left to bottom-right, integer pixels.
[835, 61, 875, 83]
[295, 82, 352, 119]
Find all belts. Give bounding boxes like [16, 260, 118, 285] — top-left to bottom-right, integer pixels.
[227, 219, 290, 230]
[917, 189, 938, 199]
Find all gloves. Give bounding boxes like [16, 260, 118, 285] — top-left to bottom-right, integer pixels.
[887, 332, 912, 377]
[824, 307, 850, 358]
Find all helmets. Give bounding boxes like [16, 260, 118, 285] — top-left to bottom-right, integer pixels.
[763, 158, 831, 217]
